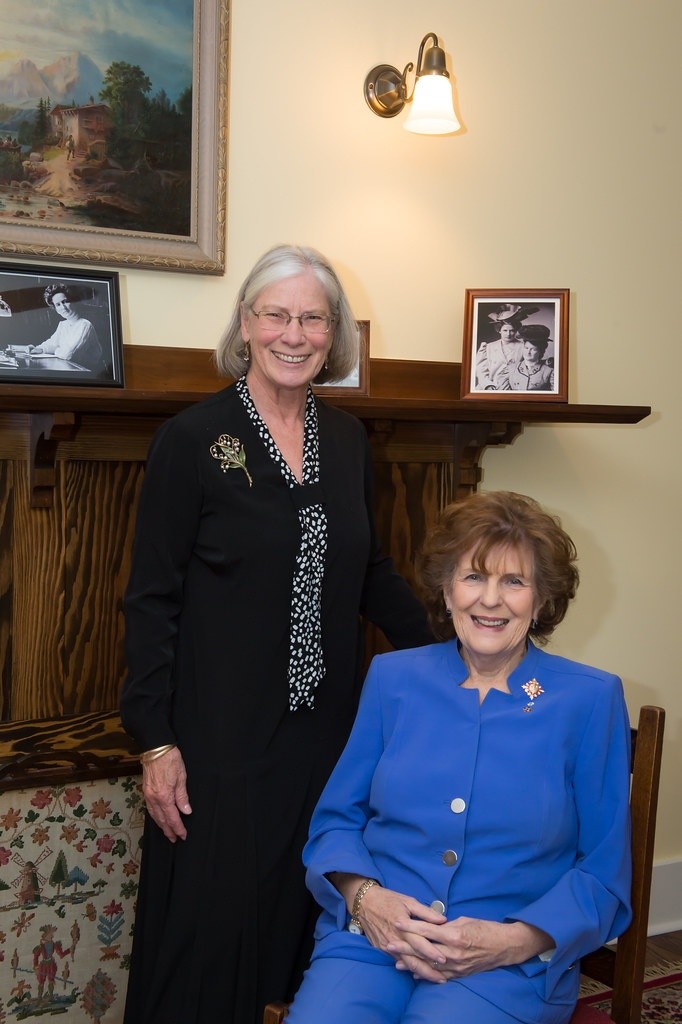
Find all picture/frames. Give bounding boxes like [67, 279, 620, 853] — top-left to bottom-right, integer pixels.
[461, 288, 570, 403]
[0, 0, 232, 276]
[309, 319, 371, 398]
[0, 260, 126, 389]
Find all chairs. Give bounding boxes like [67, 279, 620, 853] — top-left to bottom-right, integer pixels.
[264, 702, 665, 1024]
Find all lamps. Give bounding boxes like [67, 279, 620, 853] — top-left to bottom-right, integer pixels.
[363, 33, 461, 135]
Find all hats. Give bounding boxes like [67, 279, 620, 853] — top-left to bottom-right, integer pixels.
[517, 324, 550, 342]
[487, 305, 523, 324]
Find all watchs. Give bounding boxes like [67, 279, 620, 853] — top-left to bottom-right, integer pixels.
[348, 879, 379, 934]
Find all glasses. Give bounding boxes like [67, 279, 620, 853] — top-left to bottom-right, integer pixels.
[248, 304, 339, 334]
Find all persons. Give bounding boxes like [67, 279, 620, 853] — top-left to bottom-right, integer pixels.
[30, 281, 108, 378]
[116, 242, 435, 1024]
[475, 304, 555, 392]
[282, 490, 634, 1024]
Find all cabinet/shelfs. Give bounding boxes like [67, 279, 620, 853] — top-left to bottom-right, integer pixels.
[1, 343, 652, 793]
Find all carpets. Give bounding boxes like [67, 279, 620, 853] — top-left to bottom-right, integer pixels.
[577, 958, 682, 1024]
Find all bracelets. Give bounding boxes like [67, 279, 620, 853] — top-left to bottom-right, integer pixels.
[138, 744, 174, 764]
